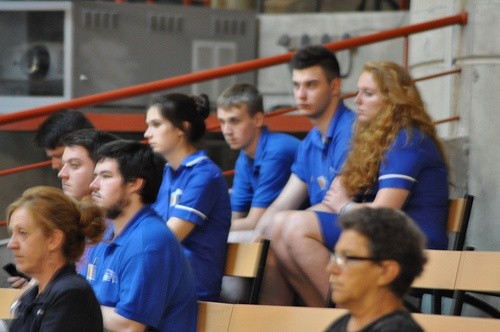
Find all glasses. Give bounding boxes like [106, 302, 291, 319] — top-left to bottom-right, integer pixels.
[328, 253, 377, 267]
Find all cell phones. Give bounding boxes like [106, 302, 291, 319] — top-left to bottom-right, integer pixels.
[3, 263, 30, 281]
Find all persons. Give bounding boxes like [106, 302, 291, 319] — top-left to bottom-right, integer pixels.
[257, 45, 362, 308]
[322, 205, 427, 332]
[56, 129, 123, 275]
[31, 109, 98, 173]
[266, 60, 450, 308]
[5, 186, 104, 332]
[79, 137, 199, 332]
[143, 92, 234, 301]
[218, 83, 302, 305]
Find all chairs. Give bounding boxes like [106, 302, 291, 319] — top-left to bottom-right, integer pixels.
[0, 195, 500, 332]
